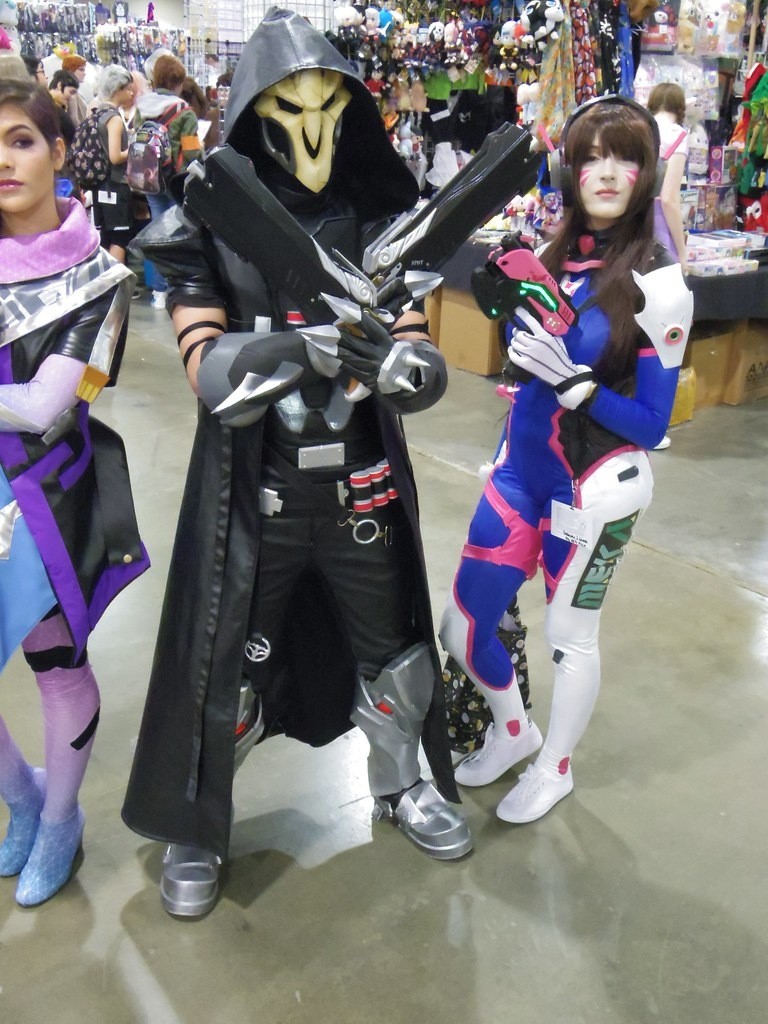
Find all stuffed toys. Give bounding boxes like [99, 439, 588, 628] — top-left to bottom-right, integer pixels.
[501, 196, 535, 234]
[632, 0, 745, 175]
[333, 0, 569, 187]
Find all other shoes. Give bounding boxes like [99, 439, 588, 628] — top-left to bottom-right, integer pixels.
[159, 842, 219, 915]
[653, 436, 671, 450]
[150, 289, 169, 309]
[131, 291, 141, 300]
[0, 767, 48, 878]
[15, 803, 86, 907]
[372, 780, 473, 860]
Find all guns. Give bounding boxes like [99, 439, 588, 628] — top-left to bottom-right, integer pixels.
[469, 231, 580, 382]
[180, 145, 378, 437]
[274, 122, 541, 433]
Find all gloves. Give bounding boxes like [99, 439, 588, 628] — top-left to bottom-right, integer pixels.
[506, 306, 593, 411]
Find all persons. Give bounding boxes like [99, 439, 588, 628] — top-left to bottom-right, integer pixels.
[122, 5, 475, 916]
[0, 28, 235, 309]
[0, 76, 152, 907]
[440, 81, 694, 822]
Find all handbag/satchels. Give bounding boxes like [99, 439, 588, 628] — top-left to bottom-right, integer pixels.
[443, 593, 532, 753]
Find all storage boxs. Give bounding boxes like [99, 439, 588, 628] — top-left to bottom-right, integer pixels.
[421, 228, 768, 429]
[680, 144, 740, 231]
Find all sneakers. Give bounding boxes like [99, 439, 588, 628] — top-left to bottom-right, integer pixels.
[496, 763, 574, 824]
[454, 718, 543, 787]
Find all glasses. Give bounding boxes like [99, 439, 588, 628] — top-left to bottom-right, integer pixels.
[33, 69, 45, 76]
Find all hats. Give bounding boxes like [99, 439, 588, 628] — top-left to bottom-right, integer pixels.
[63, 54, 88, 73]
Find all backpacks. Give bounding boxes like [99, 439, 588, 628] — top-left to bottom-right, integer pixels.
[124, 100, 196, 196]
[649, 131, 687, 198]
[65, 106, 127, 190]
